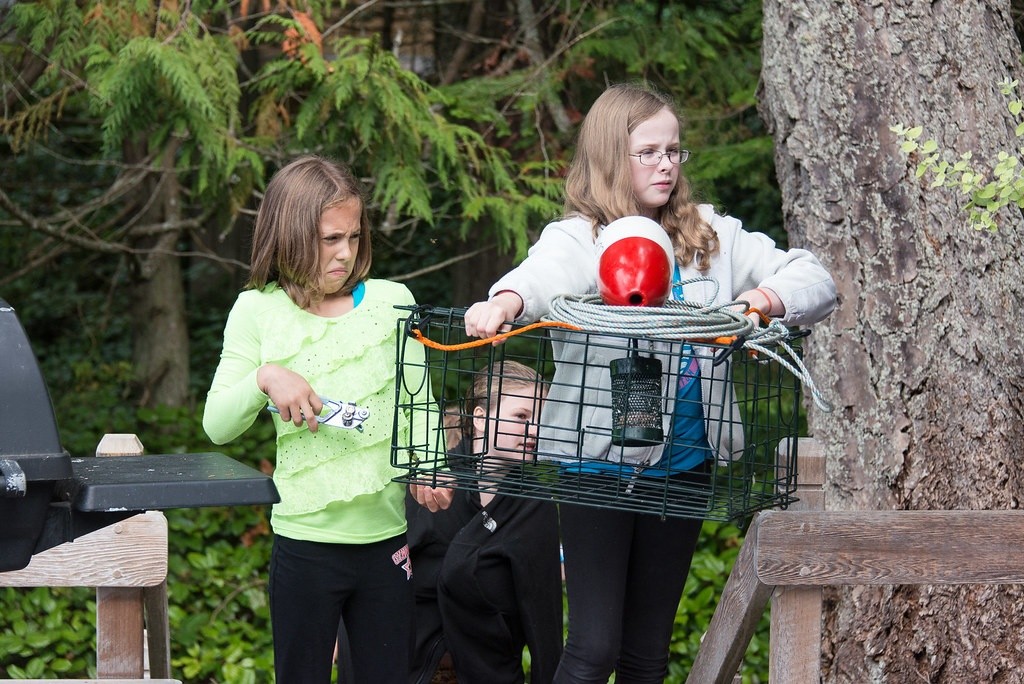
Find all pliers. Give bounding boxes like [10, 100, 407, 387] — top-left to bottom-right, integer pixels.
[266, 393, 371, 434]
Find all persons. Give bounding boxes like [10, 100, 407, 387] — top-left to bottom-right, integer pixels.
[200, 154, 462, 684]
[397, 360, 565, 683]
[461, 82, 835, 684]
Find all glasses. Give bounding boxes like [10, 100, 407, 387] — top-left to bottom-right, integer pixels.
[628, 147, 692, 167]
